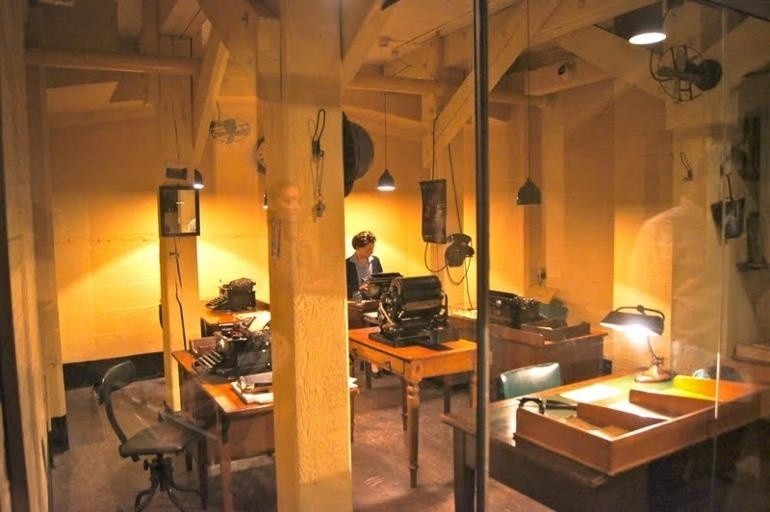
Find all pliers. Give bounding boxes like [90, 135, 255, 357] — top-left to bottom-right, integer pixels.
[546, 399, 577, 409]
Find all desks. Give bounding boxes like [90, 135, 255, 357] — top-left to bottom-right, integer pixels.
[453, 308, 610, 397]
[346, 325, 492, 487]
[171, 349, 359, 512]
[348, 300, 383, 330]
[198, 297, 271, 337]
[441, 371, 770, 512]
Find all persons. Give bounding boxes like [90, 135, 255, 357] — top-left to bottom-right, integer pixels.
[345, 229, 396, 379]
[263, 180, 304, 226]
[612, 119, 770, 511]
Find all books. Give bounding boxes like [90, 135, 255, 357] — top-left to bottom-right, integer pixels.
[230, 369, 274, 405]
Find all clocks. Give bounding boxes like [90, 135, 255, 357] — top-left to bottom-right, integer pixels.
[256, 137, 265, 175]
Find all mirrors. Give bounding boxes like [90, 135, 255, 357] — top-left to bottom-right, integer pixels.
[159, 184, 200, 237]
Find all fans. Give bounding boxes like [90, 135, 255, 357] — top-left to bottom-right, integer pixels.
[658, 47, 722, 101]
[210, 117, 250, 146]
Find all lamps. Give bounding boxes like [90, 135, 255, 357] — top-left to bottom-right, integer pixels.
[517, 1, 541, 205]
[600, 304, 674, 382]
[193, 169, 204, 189]
[377, 56, 396, 192]
[626, 0, 666, 47]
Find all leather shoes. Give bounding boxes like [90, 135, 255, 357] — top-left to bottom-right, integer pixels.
[370, 368, 393, 377]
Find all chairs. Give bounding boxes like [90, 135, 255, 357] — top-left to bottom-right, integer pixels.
[98, 359, 209, 512]
[496, 360, 563, 400]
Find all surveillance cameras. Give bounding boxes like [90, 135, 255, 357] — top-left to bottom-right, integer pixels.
[555, 62, 574, 80]
